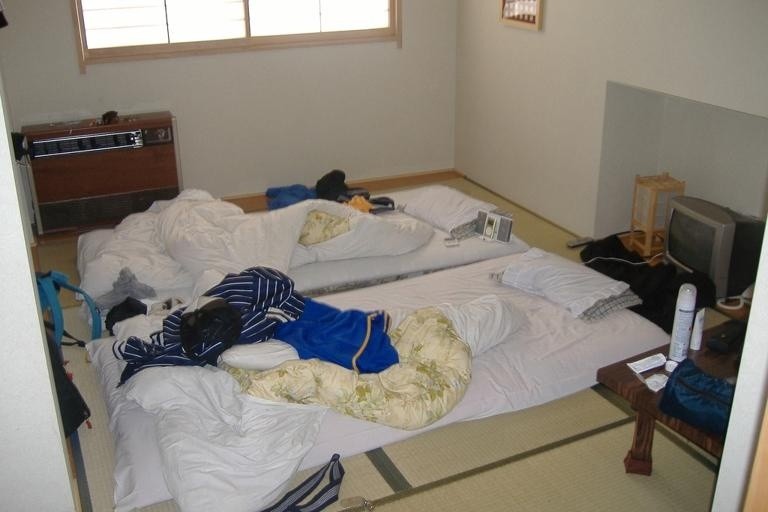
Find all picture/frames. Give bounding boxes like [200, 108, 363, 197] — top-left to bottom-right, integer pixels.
[496, 1, 542, 32]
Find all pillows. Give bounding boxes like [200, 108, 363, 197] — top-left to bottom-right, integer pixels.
[493, 247, 643, 324]
[397, 184, 499, 241]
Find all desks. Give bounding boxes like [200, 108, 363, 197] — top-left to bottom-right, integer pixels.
[597, 319, 749, 476]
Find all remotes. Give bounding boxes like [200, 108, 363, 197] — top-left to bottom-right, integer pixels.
[566, 237, 593, 248]
[450, 219, 476, 241]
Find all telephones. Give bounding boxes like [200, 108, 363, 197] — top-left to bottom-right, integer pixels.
[740, 282, 755, 304]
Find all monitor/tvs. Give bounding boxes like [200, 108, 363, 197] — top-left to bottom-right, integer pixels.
[663, 194, 765, 300]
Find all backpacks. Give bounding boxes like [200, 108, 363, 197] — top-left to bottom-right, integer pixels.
[34, 270, 103, 437]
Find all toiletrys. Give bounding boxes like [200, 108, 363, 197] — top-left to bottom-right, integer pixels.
[689, 308, 706, 351]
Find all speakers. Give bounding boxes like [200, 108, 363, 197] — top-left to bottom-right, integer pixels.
[476, 210, 487, 236]
[498, 217, 513, 245]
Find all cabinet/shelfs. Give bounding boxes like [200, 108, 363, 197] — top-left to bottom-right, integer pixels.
[629, 171, 686, 258]
